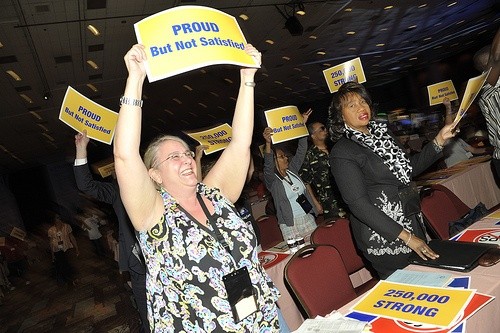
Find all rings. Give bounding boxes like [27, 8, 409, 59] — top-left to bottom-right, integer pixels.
[421, 249, 427, 254]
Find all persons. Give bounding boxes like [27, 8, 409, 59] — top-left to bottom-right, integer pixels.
[0, 228, 16, 300]
[329, 81, 460, 280]
[194, 144, 263, 254]
[298, 120, 352, 222]
[386, 119, 445, 160]
[328, 93, 345, 142]
[0, 217, 28, 283]
[473, 29, 500, 187]
[72, 129, 150, 333]
[262, 108, 318, 243]
[436, 98, 495, 168]
[113, 42, 283, 333]
[81, 209, 108, 261]
[47, 214, 83, 292]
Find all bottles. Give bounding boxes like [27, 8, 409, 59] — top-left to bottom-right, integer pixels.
[295, 233, 306, 250]
[286, 236, 299, 255]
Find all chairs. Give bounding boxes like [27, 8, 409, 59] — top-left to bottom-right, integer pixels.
[466, 136, 487, 149]
[255, 216, 283, 250]
[417, 184, 478, 241]
[310, 219, 380, 296]
[256, 184, 266, 199]
[284, 244, 358, 320]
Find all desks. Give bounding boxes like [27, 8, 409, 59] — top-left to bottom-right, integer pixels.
[291, 209, 500, 332]
[257, 150, 500, 333]
[248, 195, 268, 221]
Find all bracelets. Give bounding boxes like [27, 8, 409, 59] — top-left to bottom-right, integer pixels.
[240, 82, 255, 87]
[119, 96, 144, 107]
[405, 230, 412, 246]
[445, 112, 452, 116]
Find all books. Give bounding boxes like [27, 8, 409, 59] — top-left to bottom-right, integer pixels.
[408, 238, 498, 273]
